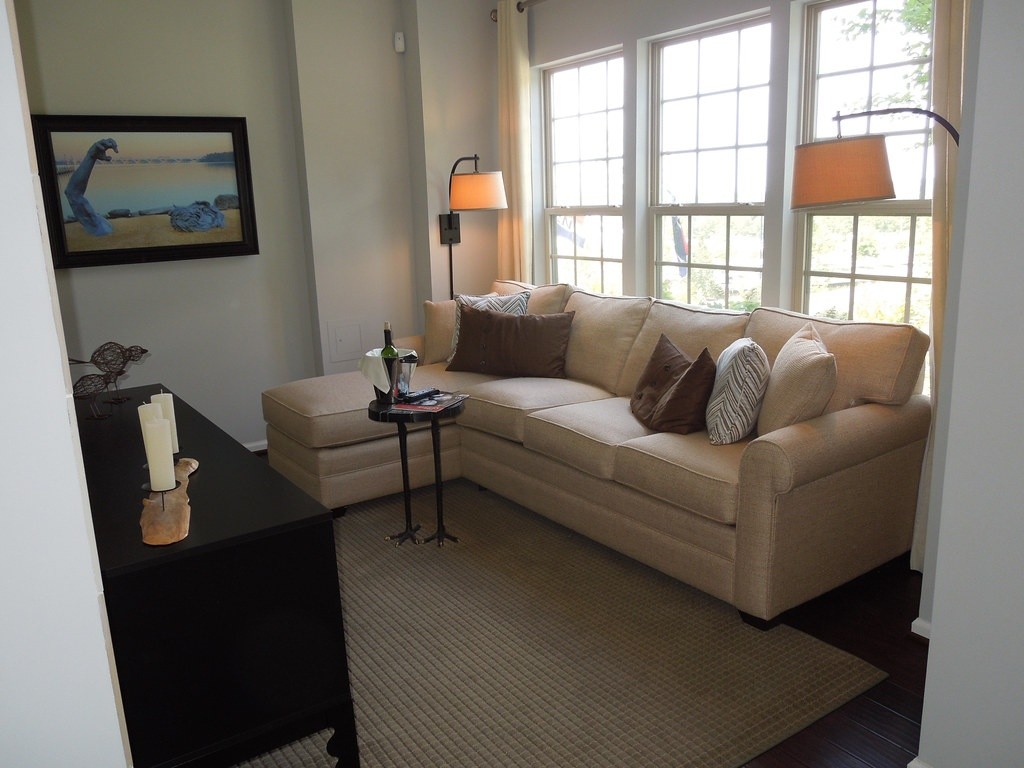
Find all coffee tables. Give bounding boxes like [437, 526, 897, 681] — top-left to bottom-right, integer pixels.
[369, 390, 460, 548]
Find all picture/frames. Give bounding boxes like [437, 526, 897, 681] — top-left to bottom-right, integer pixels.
[30, 115, 261, 270]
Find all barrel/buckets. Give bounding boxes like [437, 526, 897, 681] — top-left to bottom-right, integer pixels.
[366, 348, 419, 404]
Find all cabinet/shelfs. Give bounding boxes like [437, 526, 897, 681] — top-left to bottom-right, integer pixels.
[69, 383, 360, 768]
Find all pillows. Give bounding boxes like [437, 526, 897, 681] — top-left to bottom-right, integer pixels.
[745, 307, 932, 411]
[424, 299, 455, 362]
[618, 299, 752, 398]
[492, 278, 575, 315]
[444, 304, 576, 379]
[446, 290, 532, 361]
[705, 337, 771, 445]
[630, 333, 717, 435]
[756, 322, 838, 438]
[563, 290, 656, 394]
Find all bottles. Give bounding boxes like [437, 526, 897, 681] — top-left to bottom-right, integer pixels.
[381, 321, 398, 357]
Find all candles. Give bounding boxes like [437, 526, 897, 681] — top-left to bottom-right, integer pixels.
[150, 389, 180, 454]
[147, 414, 177, 491]
[138, 401, 163, 460]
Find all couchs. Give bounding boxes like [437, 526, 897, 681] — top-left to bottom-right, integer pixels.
[256, 362, 934, 629]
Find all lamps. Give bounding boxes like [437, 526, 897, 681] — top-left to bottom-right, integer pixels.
[790, 108, 959, 210]
[440, 153, 510, 299]
